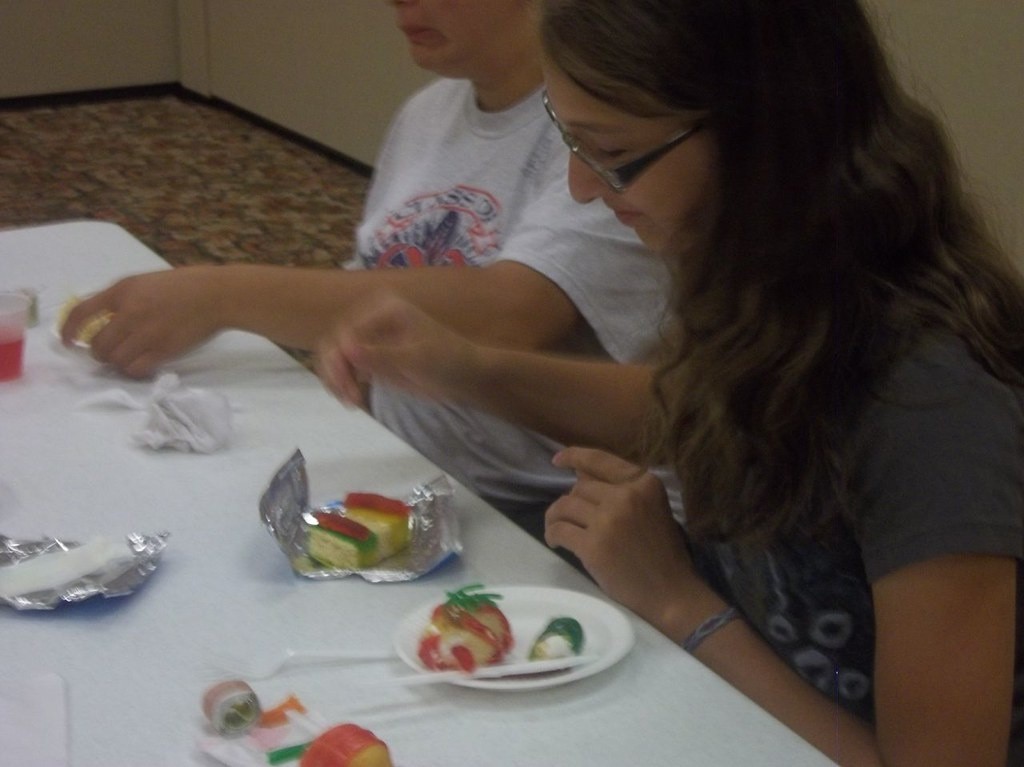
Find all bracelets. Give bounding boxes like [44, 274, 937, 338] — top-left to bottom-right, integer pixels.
[681, 607, 745, 653]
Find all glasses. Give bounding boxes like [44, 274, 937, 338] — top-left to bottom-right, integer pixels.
[542, 88, 704, 193]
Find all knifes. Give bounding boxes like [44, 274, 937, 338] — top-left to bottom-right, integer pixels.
[388, 654, 596, 687]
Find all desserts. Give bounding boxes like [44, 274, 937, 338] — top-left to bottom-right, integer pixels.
[298, 723, 394, 767]
[307, 493, 413, 571]
[417, 584, 583, 673]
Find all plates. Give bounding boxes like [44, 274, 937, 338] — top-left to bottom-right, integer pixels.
[394, 584, 635, 690]
[51, 291, 113, 350]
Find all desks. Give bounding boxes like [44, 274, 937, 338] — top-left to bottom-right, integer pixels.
[0, 221, 840, 767]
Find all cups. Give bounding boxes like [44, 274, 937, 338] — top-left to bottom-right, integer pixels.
[0, 289, 31, 381]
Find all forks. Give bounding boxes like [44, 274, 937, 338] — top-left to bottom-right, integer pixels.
[203, 644, 393, 679]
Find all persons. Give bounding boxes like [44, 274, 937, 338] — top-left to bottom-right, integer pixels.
[59, 0, 686, 551]
[322, 0, 1024, 767]
